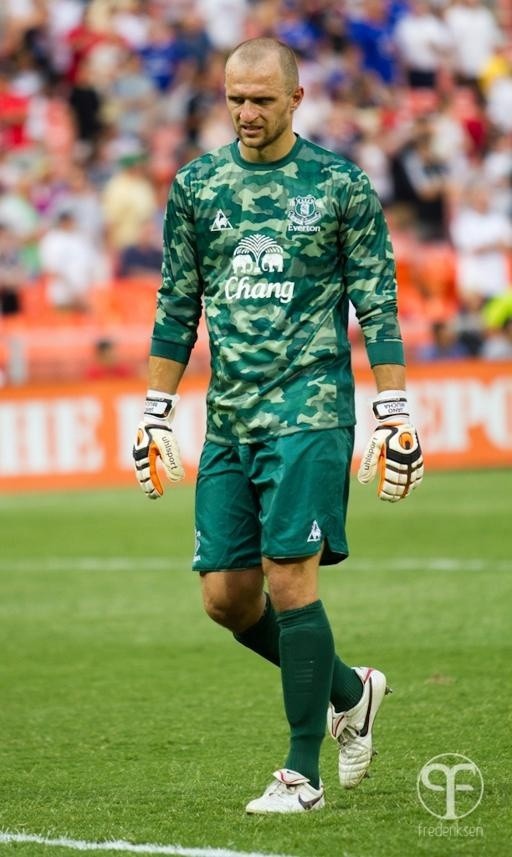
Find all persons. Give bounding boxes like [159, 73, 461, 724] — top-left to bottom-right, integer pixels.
[1, 0, 512, 364]
[133, 36, 426, 817]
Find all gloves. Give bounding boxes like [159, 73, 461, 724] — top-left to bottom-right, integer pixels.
[358, 392, 424, 503]
[131, 390, 184, 500]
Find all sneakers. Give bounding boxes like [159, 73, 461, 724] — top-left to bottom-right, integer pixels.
[329, 667, 388, 787]
[244, 770, 326, 816]
